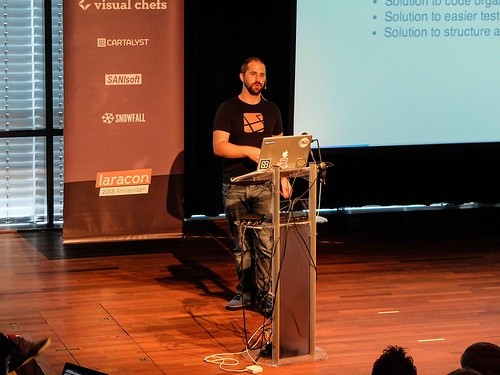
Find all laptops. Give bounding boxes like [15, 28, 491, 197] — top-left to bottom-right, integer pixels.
[256, 135, 312, 171]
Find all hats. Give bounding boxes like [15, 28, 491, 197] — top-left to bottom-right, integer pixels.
[7, 335, 49, 373]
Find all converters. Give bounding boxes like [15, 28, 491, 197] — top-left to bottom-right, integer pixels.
[246, 365, 263, 373]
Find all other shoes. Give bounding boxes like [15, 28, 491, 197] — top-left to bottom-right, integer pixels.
[255, 294, 273, 314]
[227, 294, 252, 308]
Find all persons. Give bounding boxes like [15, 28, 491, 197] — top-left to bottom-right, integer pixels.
[213, 56, 293, 317]
[447, 368, 481, 375]
[0, 331, 51, 375]
[461, 342, 500, 375]
[371, 346, 417, 375]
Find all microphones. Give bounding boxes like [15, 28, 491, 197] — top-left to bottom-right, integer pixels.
[265, 83, 266, 89]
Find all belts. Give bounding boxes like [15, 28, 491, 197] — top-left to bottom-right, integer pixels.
[230, 181, 265, 185]
